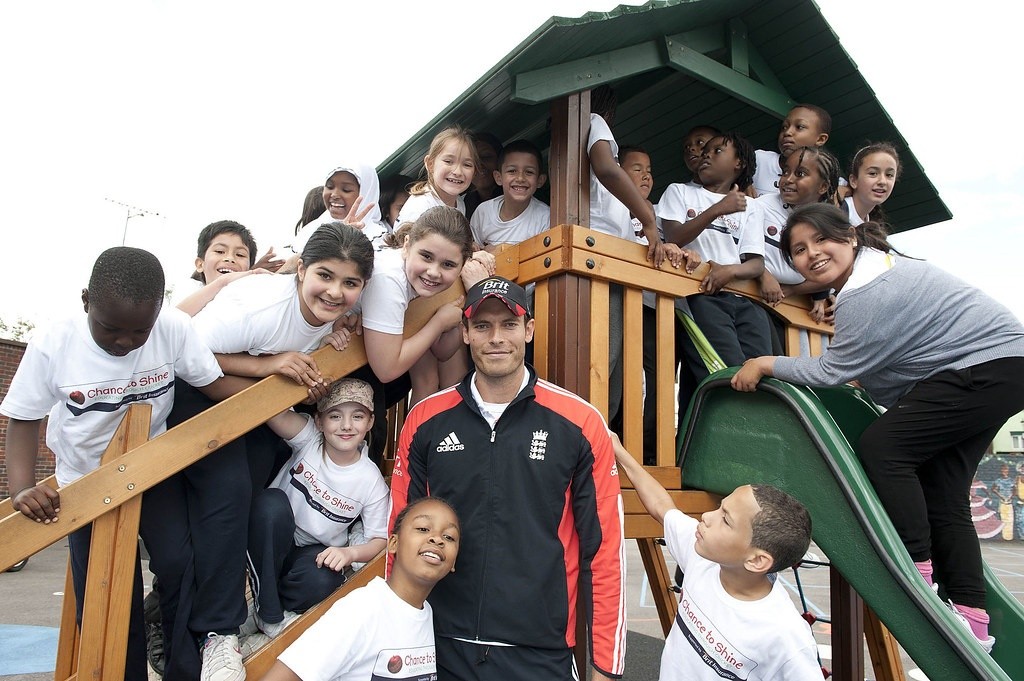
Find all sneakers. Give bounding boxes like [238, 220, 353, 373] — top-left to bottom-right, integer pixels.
[238, 603, 259, 637]
[145, 620, 165, 675]
[237, 632, 272, 659]
[201, 631, 246, 681]
[253, 608, 301, 638]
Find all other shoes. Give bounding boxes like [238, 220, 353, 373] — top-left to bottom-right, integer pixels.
[944, 599, 995, 654]
[929, 582, 939, 595]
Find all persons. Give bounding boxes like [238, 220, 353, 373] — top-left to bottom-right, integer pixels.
[585, 82, 899, 465]
[261, 495, 461, 681]
[610, 430, 825, 681]
[0, 128, 550, 681]
[385, 275, 627, 681]
[730, 202, 1024, 654]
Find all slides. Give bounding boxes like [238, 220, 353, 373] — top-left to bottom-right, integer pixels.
[676, 365, 1024, 681]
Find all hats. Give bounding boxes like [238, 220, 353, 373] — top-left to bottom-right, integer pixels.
[462, 276, 530, 320]
[316, 377, 375, 413]
